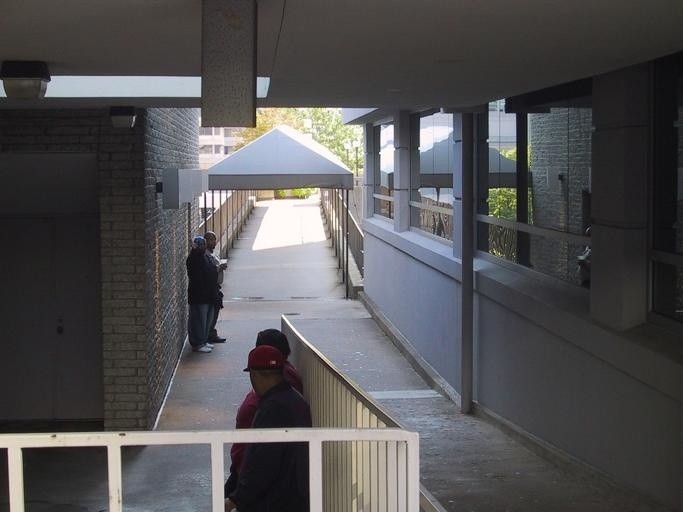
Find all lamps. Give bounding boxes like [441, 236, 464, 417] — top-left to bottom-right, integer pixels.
[110, 107, 137, 128]
[0, 61, 51, 102]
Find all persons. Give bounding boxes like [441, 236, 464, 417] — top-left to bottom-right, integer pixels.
[223, 343, 313, 511]
[224, 329, 305, 494]
[201, 228, 227, 345]
[186, 235, 216, 353]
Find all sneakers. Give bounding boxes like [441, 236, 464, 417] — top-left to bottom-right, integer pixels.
[197, 336, 226, 352]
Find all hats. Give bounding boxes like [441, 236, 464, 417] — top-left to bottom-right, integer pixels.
[243, 329, 291, 371]
